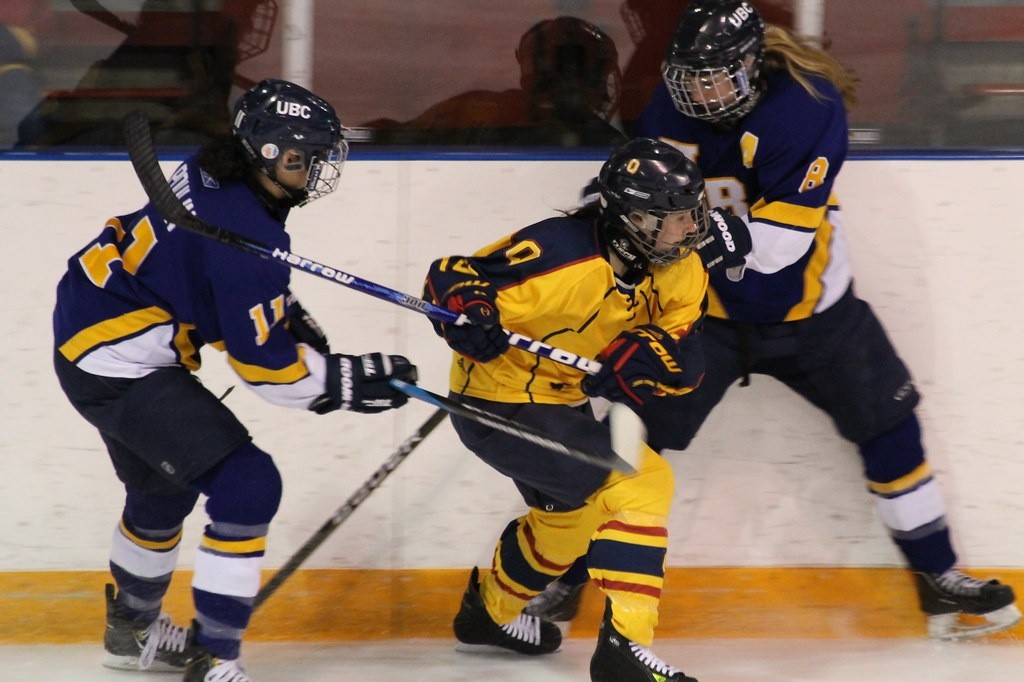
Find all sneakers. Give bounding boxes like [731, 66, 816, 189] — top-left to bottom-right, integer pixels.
[590, 596, 697, 682]
[904, 563, 1022, 640]
[521, 577, 589, 636]
[180, 618, 249, 682]
[453, 565, 563, 654]
[102, 583, 194, 672]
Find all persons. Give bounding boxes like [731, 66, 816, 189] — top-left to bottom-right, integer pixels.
[522, 1, 1024, 639]
[421, 137, 732, 681]
[52, 78, 420, 681]
[363, 0, 831, 148]
[0, 1, 278, 153]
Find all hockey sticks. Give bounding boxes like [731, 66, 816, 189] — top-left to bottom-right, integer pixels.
[119, 110, 608, 376]
[389, 380, 645, 476]
[251, 404, 449, 607]
[72, 0, 258, 90]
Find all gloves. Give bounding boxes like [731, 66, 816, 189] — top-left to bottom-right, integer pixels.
[440, 280, 510, 363]
[284, 289, 331, 356]
[678, 207, 752, 288]
[307, 352, 419, 415]
[579, 322, 681, 408]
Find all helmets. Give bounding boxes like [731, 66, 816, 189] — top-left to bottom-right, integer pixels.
[598, 137, 710, 266]
[661, 0, 765, 122]
[620, 0, 690, 73]
[229, 78, 349, 209]
[135, 0, 278, 67]
[512, 16, 623, 124]
